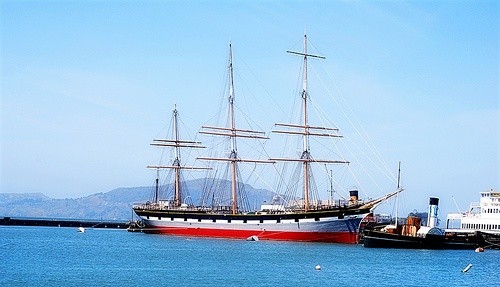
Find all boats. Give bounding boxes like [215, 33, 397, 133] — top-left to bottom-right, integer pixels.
[446, 189, 500, 236]
[127, 220, 142, 233]
[362, 195, 485, 252]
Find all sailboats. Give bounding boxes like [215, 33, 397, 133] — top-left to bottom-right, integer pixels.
[129, 29, 406, 244]
